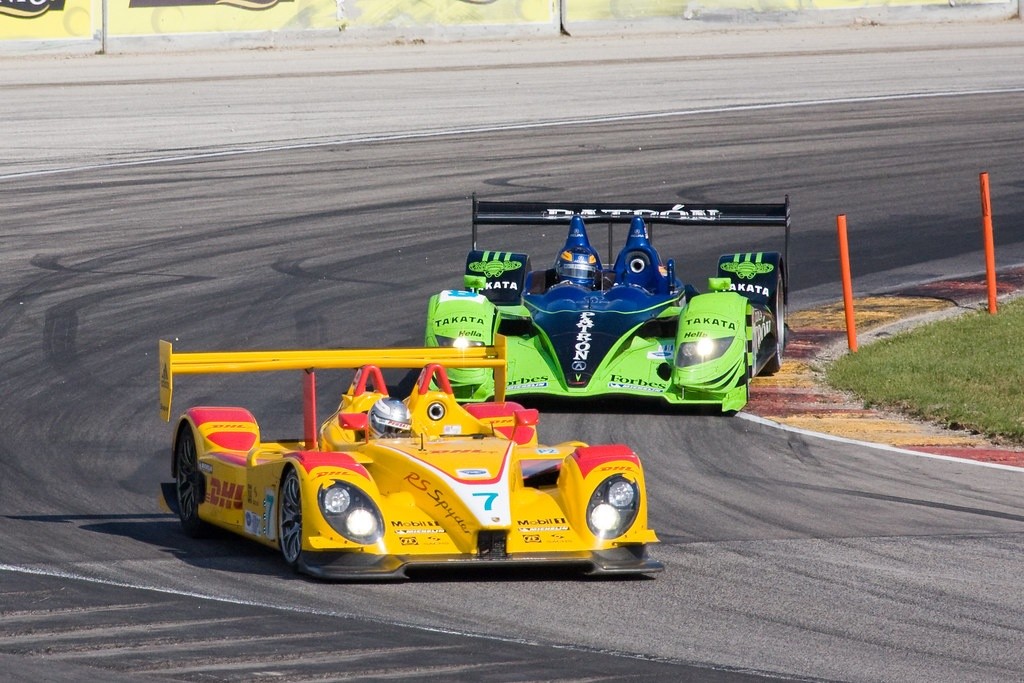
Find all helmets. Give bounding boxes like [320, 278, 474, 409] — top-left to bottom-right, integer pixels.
[555, 246, 598, 287]
[367, 397, 412, 440]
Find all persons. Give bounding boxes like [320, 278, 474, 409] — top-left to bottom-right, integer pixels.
[365, 396, 412, 442]
[552, 242, 604, 292]
